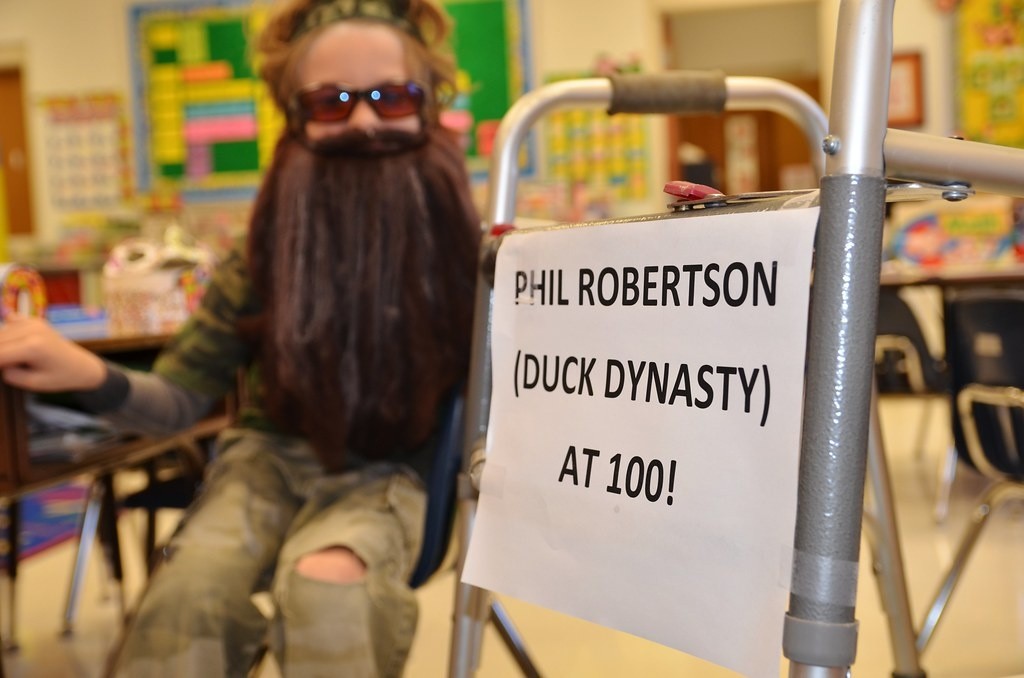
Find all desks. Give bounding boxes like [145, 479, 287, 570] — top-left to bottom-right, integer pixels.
[1, 324, 219, 650]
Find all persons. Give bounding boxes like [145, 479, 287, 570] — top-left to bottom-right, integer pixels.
[0, 1, 486, 678]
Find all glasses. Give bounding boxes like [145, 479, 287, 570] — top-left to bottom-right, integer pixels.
[284, 78, 427, 122]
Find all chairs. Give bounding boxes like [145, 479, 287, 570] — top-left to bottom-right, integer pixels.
[918, 276, 1023, 655]
[406, 390, 538, 676]
